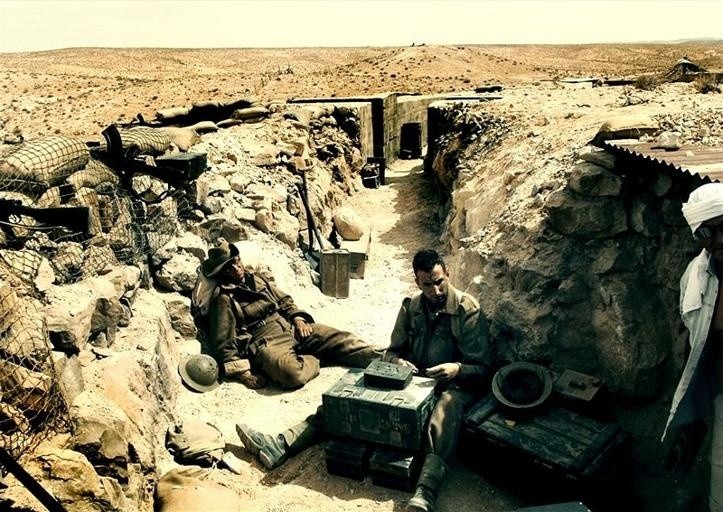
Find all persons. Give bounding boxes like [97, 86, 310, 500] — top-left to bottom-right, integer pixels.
[234, 246, 498, 512]
[662, 179, 722, 511]
[199, 239, 392, 389]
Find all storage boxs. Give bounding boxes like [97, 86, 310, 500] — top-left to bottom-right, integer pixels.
[320, 249, 351, 299]
[324, 370, 439, 450]
[460, 397, 624, 504]
[325, 437, 377, 482]
[368, 443, 428, 493]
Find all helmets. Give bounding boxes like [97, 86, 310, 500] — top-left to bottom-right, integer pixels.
[491, 361, 552, 410]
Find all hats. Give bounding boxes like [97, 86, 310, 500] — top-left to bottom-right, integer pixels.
[203, 241, 239, 276]
[179, 354, 220, 392]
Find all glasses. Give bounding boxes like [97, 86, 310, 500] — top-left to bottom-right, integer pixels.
[418, 278, 444, 288]
[696, 225, 722, 239]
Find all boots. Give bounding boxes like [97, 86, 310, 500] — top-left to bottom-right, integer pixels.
[236, 423, 290, 469]
[407, 485, 435, 511]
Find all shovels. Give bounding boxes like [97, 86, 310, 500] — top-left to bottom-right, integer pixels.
[302, 170, 321, 272]
